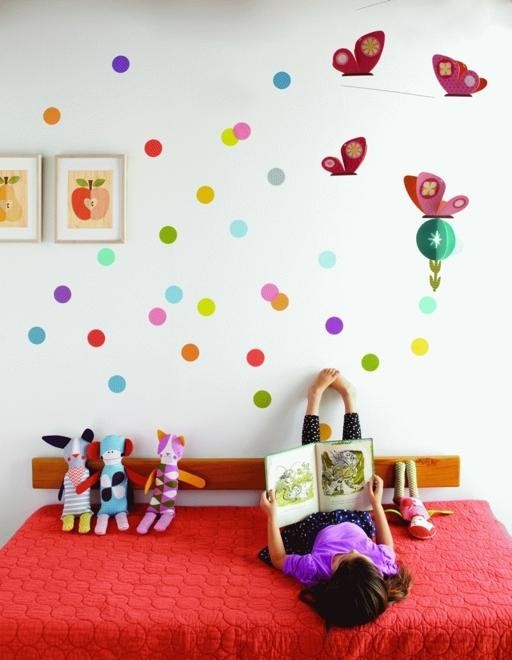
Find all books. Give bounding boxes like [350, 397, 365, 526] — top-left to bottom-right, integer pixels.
[264, 438, 373, 529]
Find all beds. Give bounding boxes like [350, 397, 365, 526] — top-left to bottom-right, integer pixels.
[0, 455, 511, 660]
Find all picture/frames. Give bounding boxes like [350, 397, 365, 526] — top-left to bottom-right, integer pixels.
[51, 152, 126, 242]
[0, 152, 41, 241]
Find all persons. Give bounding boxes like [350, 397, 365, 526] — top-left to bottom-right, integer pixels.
[258, 368, 415, 628]
